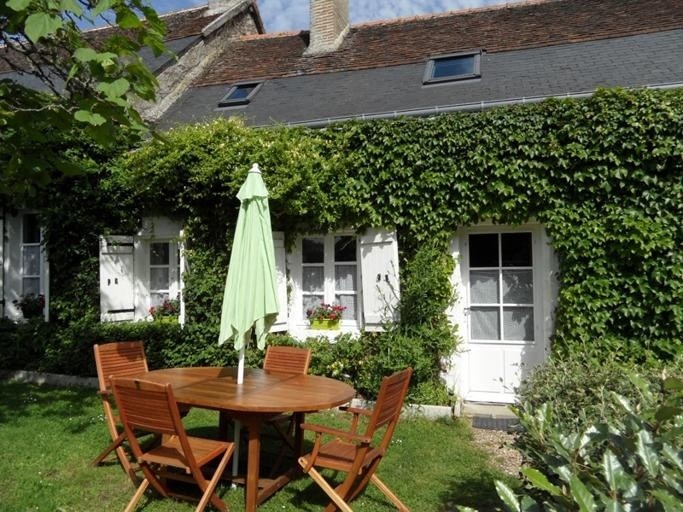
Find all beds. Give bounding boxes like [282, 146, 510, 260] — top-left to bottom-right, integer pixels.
[218, 160, 278, 490]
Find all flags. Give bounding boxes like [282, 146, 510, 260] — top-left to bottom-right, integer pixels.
[21, 308, 42, 318]
[154, 316, 178, 323]
[309, 318, 340, 330]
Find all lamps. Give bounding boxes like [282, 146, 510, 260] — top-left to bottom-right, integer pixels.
[89, 340, 190, 484]
[298, 367, 414, 512]
[108, 375, 237, 512]
[217, 345, 311, 472]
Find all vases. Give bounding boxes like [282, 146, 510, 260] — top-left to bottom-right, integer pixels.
[149, 299, 180, 321]
[306, 302, 347, 324]
[13, 293, 44, 308]
